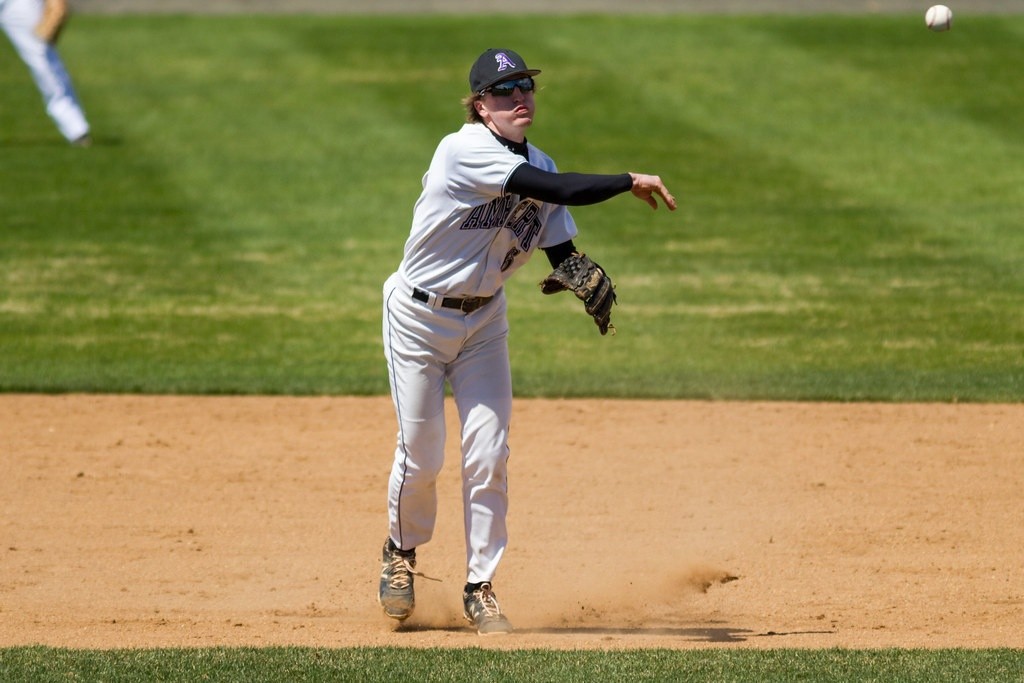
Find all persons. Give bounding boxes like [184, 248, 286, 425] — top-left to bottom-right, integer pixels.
[380, 47, 677, 634]
[0, 0, 94, 150]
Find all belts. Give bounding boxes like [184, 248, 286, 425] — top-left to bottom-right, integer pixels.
[413, 288, 494, 314]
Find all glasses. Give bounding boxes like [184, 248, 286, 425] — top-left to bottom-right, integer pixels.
[480, 76, 535, 97]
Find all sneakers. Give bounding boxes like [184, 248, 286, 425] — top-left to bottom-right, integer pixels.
[462, 584, 514, 635]
[378, 535, 426, 620]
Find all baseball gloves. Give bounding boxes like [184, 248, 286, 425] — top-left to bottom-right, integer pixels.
[537, 250, 619, 336]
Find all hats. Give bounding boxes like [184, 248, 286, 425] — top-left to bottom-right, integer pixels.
[469, 48, 542, 94]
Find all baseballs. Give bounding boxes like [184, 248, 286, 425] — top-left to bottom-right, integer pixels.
[924, 5, 954, 32]
